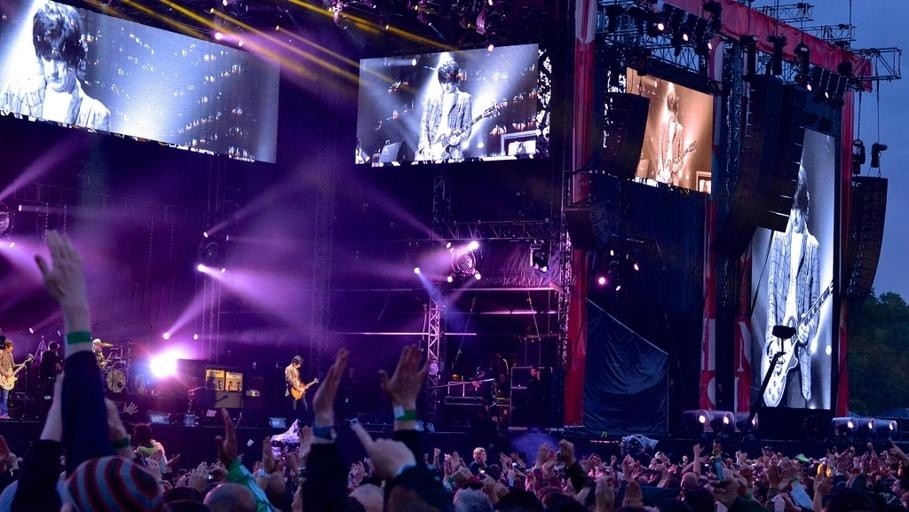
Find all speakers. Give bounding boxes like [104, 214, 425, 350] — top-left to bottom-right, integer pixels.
[510, 366, 556, 426]
[200, 390, 244, 408]
[726, 79, 808, 231]
[599, 92, 650, 180]
[839, 178, 888, 301]
[565, 198, 612, 252]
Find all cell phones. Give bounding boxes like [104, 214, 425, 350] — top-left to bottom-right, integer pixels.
[711, 458, 724, 488]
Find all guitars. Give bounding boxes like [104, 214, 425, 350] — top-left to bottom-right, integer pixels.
[414, 102, 508, 162]
[0, 357, 31, 390]
[761, 278, 833, 407]
[289, 377, 320, 401]
[658, 140, 698, 184]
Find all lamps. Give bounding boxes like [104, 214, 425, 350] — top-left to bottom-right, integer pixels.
[605, 0, 848, 104]
[443, 235, 489, 285]
[0, 199, 26, 252]
[192, 232, 234, 275]
[592, 246, 642, 292]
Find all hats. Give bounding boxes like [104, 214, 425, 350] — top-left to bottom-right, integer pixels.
[63, 456, 164, 511]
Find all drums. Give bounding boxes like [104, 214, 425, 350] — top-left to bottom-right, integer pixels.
[105, 368, 127, 394]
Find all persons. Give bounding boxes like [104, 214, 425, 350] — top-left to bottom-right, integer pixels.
[414, 61, 472, 157]
[762, 161, 820, 408]
[0, 0, 111, 134]
[653, 92, 684, 185]
[2, 226, 909, 511]
